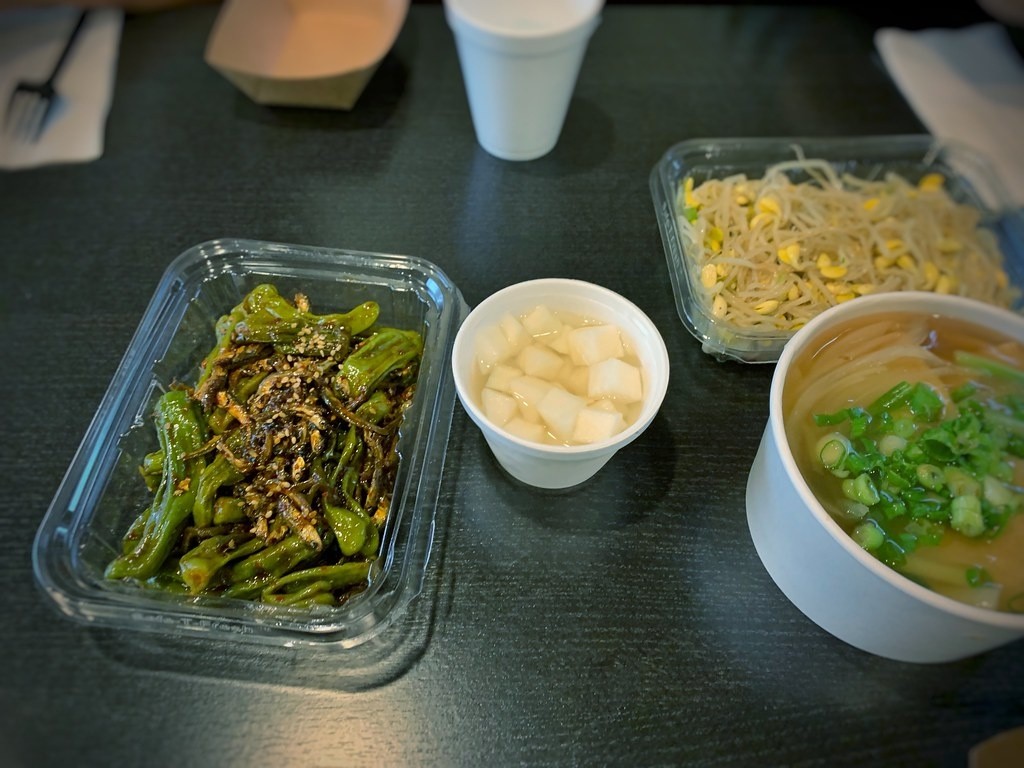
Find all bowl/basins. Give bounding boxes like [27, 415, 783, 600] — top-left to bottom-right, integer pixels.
[648, 131, 1024, 368]
[31, 237, 466, 658]
[743, 290, 1024, 666]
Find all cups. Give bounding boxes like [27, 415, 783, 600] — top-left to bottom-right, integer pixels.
[452, 276, 672, 491]
[443, 0, 606, 162]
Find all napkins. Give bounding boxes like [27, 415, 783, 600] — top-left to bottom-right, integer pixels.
[0, 4, 125, 172]
[874, 22, 1024, 221]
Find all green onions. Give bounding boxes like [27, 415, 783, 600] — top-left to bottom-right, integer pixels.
[811, 353, 1023, 588]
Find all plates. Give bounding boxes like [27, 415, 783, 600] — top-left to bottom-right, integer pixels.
[0, 0, 126, 170]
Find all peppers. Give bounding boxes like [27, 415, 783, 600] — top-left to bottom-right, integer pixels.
[107, 288, 425, 611]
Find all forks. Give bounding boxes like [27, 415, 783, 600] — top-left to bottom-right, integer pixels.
[8, 0, 98, 145]
